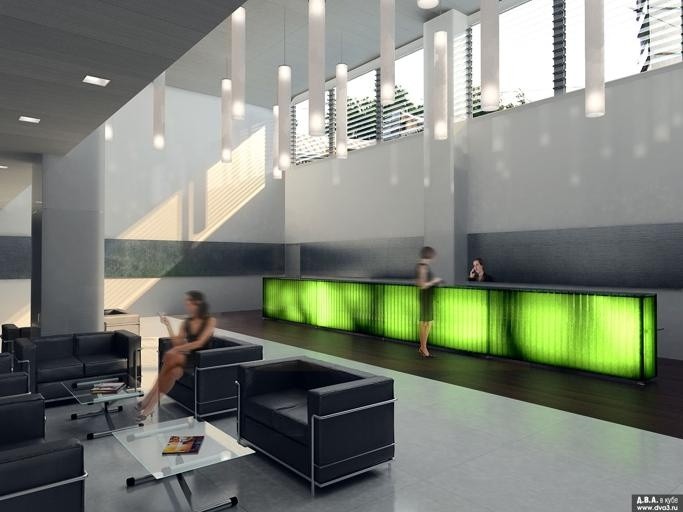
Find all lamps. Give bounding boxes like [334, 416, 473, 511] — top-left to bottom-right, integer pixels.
[106, 0, 605, 180]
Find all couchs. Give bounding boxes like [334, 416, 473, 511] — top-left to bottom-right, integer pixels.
[15, 330, 141, 409]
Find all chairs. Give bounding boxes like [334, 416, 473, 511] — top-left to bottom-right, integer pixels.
[0, 393, 88, 512]
[0, 324, 30, 353]
[234, 356, 398, 497]
[0, 353, 31, 399]
[159, 337, 263, 420]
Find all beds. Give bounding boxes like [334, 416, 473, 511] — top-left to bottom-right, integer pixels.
[61, 373, 144, 440]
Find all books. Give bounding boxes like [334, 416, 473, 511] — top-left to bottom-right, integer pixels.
[90, 382, 126, 394]
[161, 435, 206, 456]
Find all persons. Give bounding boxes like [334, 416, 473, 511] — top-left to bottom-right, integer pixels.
[467, 258, 493, 282]
[413, 246, 444, 360]
[134, 290, 216, 422]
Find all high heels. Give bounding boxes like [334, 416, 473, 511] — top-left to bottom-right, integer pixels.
[418, 347, 434, 360]
[134, 401, 154, 422]
